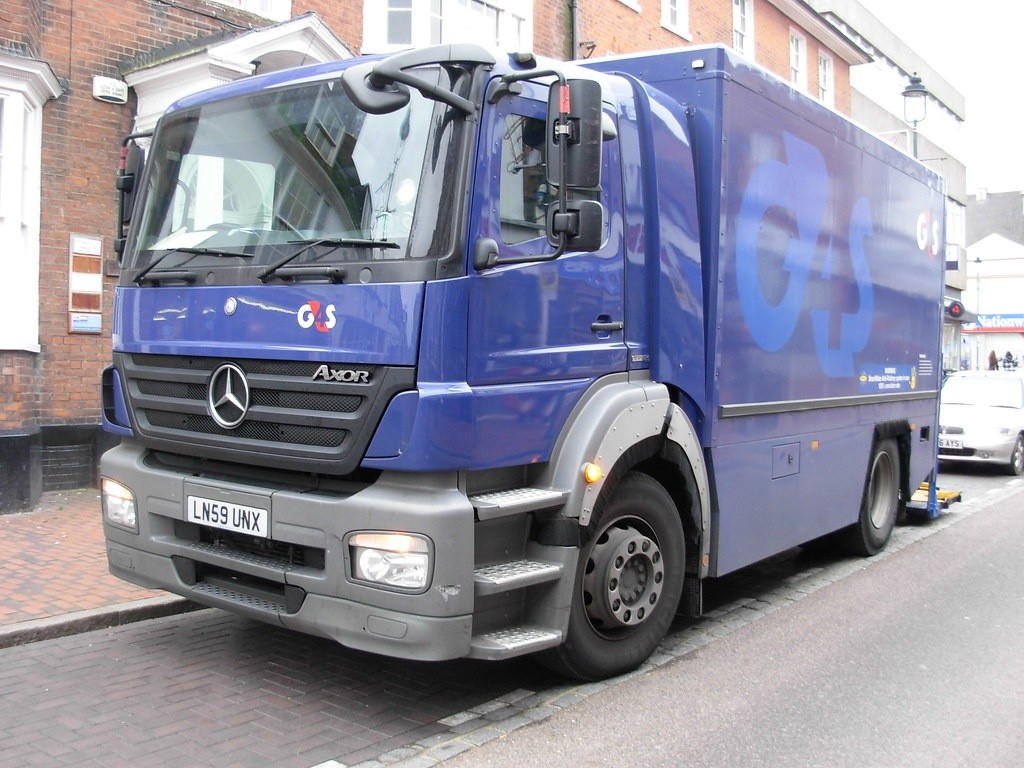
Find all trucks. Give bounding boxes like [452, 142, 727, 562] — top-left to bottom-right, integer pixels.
[98, 32, 946, 683]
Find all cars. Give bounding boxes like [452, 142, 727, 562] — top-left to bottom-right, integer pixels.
[937, 369, 1024, 476]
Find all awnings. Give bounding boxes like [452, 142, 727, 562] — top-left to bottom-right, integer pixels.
[944, 306, 979, 324]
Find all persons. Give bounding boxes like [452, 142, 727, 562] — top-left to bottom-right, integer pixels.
[988, 350, 999, 371]
